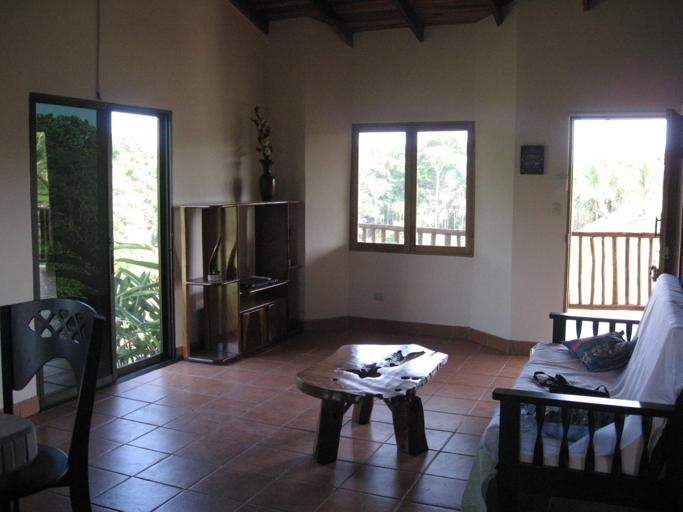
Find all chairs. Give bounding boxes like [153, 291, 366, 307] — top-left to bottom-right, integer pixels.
[0, 296, 107, 511]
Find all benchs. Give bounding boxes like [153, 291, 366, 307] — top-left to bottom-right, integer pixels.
[474, 274, 680, 508]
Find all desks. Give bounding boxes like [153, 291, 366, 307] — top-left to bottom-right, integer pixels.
[0, 414, 37, 474]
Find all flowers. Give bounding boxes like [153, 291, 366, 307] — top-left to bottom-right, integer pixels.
[249, 103, 276, 160]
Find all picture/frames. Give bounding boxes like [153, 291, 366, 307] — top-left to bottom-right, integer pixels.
[519, 145, 545, 176]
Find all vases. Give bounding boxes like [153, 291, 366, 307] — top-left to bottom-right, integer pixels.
[257, 158, 277, 202]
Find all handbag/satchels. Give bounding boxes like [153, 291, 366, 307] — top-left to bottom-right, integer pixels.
[534, 370, 610, 398]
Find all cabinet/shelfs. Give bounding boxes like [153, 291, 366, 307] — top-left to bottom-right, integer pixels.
[177, 196, 303, 366]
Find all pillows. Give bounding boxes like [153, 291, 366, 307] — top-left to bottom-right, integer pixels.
[517, 387, 621, 428]
[563, 328, 638, 374]
